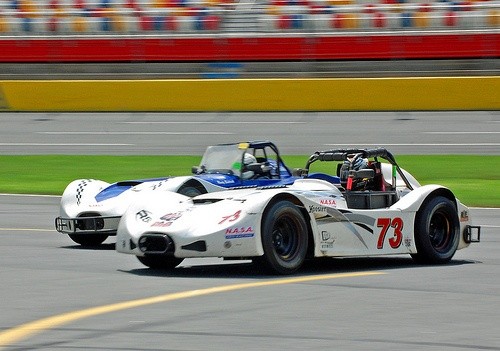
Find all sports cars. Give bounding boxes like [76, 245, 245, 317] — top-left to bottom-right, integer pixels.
[51, 140, 344, 260]
[111, 137, 481, 275]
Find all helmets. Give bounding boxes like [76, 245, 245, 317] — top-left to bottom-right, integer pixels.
[232, 153, 256, 179]
[340, 157, 370, 191]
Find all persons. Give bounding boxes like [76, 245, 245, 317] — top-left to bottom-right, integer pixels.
[340, 156, 369, 190]
[231, 152, 258, 179]
